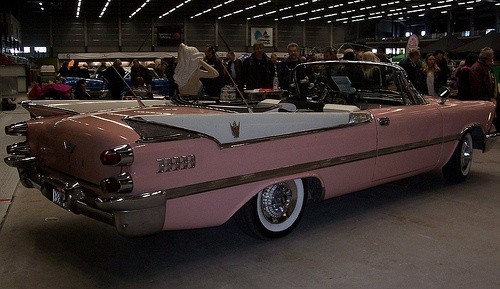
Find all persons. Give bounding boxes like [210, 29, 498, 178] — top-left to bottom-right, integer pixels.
[270, 42, 388, 91]
[399, 47, 496, 101]
[56, 58, 155, 100]
[240, 42, 275, 90]
[155, 43, 241, 99]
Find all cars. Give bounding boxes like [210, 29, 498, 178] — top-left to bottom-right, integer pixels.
[60, 66, 171, 100]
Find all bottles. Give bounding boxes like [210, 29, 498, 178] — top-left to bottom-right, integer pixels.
[220, 85, 236, 100]
[273, 72, 279, 90]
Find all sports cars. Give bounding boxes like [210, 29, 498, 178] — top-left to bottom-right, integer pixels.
[3, 57, 500, 242]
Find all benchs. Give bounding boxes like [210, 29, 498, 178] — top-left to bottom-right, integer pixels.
[259, 98, 358, 113]
